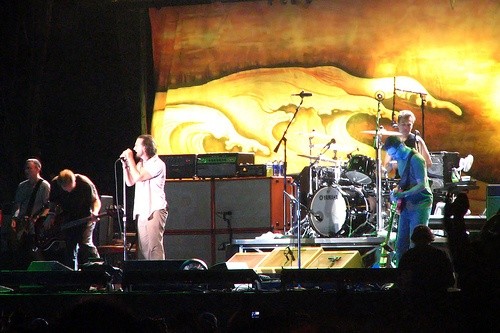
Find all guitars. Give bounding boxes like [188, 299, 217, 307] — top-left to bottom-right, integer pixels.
[34, 204, 125, 250]
[14, 200, 49, 244]
[390, 185, 404, 213]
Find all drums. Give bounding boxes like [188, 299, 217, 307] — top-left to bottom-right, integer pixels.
[309, 186, 369, 238]
[345, 154, 375, 185]
[368, 178, 396, 194]
[386, 160, 403, 181]
[315, 168, 345, 187]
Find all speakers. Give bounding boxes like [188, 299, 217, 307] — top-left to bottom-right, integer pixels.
[426, 150, 461, 194]
[91, 195, 113, 246]
[486, 184, 500, 220]
[122, 175, 366, 289]
[28, 260, 73, 270]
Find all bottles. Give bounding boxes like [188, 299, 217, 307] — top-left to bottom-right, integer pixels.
[265, 157, 285, 177]
[388, 163, 395, 179]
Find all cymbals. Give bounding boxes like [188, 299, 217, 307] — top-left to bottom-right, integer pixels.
[361, 129, 404, 135]
[296, 154, 339, 162]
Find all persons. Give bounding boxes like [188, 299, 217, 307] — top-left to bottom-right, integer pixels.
[120, 134, 171, 261]
[10, 158, 50, 281]
[381, 108, 456, 333]
[49, 168, 103, 272]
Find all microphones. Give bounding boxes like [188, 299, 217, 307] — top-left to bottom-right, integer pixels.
[314, 214, 323, 221]
[118, 150, 136, 160]
[391, 122, 398, 129]
[378, 94, 382, 98]
[287, 247, 295, 261]
[326, 139, 335, 146]
[292, 93, 313, 96]
[217, 211, 232, 215]
